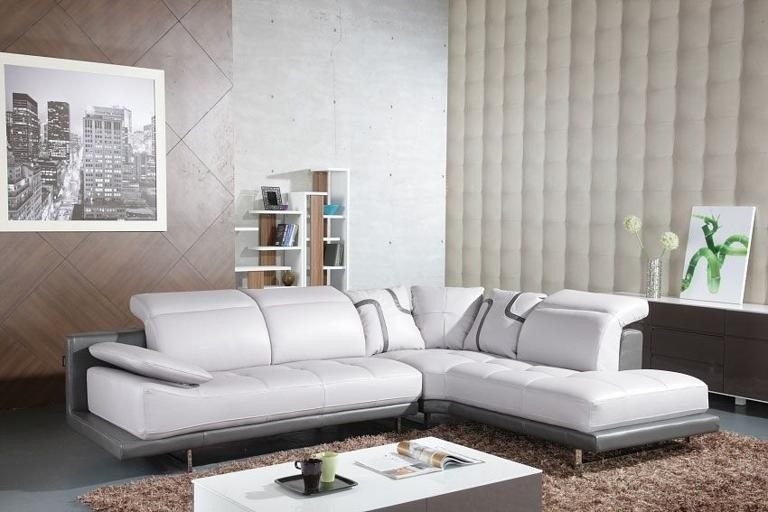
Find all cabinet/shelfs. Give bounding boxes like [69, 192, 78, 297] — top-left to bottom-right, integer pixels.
[623, 303, 768, 403]
[235, 168, 350, 289]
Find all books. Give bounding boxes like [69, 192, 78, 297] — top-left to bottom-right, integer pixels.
[274, 223, 299, 247]
[324, 243, 343, 266]
[354, 440, 485, 480]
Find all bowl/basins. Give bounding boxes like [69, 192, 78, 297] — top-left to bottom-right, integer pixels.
[324, 201, 342, 215]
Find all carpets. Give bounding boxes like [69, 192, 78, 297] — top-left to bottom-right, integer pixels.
[76, 423, 768, 512]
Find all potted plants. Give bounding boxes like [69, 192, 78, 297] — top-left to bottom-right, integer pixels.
[623, 216, 678, 259]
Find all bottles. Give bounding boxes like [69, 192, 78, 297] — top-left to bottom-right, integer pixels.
[240, 277, 248, 289]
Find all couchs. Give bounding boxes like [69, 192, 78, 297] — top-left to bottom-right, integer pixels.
[61, 329, 719, 472]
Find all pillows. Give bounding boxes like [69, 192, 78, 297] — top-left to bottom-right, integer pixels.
[89, 341, 212, 383]
[516, 288, 649, 371]
[345, 284, 425, 356]
[463, 288, 546, 360]
[238, 285, 364, 365]
[410, 285, 484, 350]
[129, 288, 271, 374]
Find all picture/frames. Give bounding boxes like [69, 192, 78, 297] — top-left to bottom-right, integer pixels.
[0, 52, 167, 232]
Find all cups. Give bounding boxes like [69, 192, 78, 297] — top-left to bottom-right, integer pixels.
[279, 201, 288, 209]
[294, 458, 322, 491]
[311, 452, 338, 483]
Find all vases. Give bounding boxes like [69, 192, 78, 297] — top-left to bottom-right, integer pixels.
[645, 256, 661, 298]
